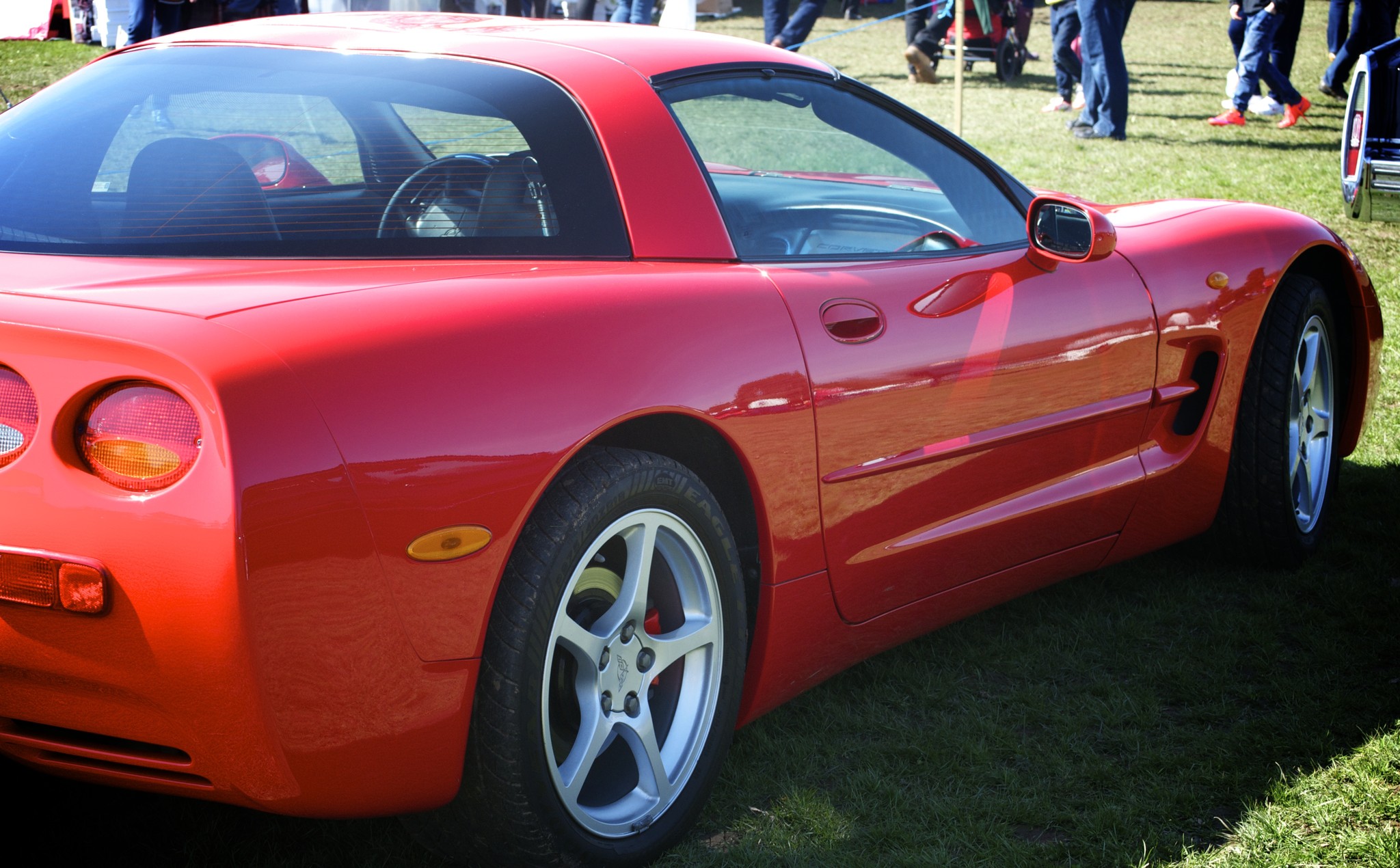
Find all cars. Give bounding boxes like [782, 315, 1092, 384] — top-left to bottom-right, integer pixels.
[0, 10, 1390, 867]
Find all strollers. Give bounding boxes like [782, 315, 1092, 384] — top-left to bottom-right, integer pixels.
[917, 0, 1034, 83]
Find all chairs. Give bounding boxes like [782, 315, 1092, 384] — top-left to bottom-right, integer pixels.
[470, 148, 558, 242]
[119, 135, 281, 243]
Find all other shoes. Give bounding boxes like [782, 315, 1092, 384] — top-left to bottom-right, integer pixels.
[1075, 128, 1127, 140]
[1065, 119, 1093, 130]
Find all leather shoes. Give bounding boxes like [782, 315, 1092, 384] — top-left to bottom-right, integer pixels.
[1318, 80, 1349, 102]
[905, 45, 937, 84]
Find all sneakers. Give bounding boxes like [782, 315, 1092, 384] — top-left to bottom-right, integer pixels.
[1278, 97, 1311, 129]
[1071, 92, 1085, 109]
[1041, 101, 1072, 112]
[1221, 94, 1263, 109]
[1207, 108, 1245, 126]
[1248, 96, 1284, 115]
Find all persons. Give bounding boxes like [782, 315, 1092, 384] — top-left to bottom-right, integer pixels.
[1202, 1, 1400, 130]
[40, 2, 669, 50]
[1039, 0, 1089, 116]
[1067, 0, 1133, 141]
[763, 0, 819, 52]
[900, 0, 959, 86]
